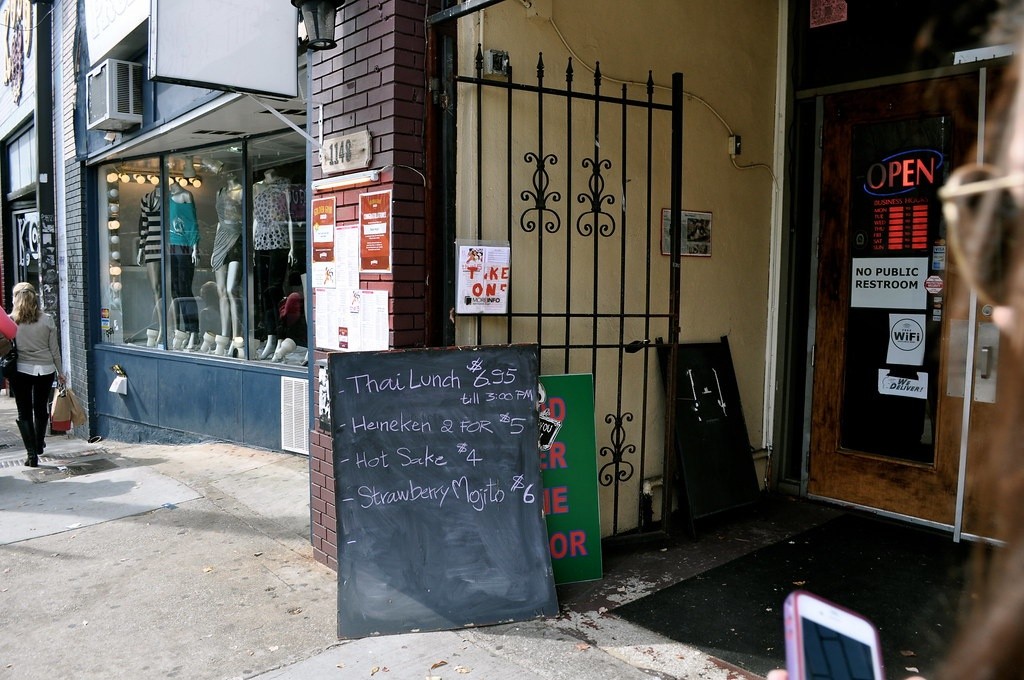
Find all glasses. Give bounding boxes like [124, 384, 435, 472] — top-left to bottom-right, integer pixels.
[938, 163, 1024, 305]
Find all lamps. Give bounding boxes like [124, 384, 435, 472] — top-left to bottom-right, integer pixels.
[291, 0, 345, 50]
[214, 334, 229, 356]
[182, 154, 196, 179]
[171, 328, 187, 351]
[274, 338, 297, 364]
[202, 153, 225, 175]
[146, 328, 158, 347]
[201, 332, 215, 354]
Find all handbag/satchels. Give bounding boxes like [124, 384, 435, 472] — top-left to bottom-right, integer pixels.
[51, 381, 87, 431]
[0, 338, 18, 377]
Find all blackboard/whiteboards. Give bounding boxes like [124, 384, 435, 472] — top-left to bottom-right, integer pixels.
[326, 342, 561, 640]
[657, 342, 762, 518]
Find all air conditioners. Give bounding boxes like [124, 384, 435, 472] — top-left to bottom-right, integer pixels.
[85, 58, 143, 133]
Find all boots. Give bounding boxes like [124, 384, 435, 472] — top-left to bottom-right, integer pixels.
[34, 413, 49, 454]
[16, 419, 38, 467]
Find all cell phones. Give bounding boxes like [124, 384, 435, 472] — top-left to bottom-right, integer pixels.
[784, 591, 885, 680]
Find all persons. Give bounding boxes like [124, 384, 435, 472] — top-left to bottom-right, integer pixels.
[0, 281, 66, 469]
[765, 6, 1024, 680]
[136, 168, 307, 366]
